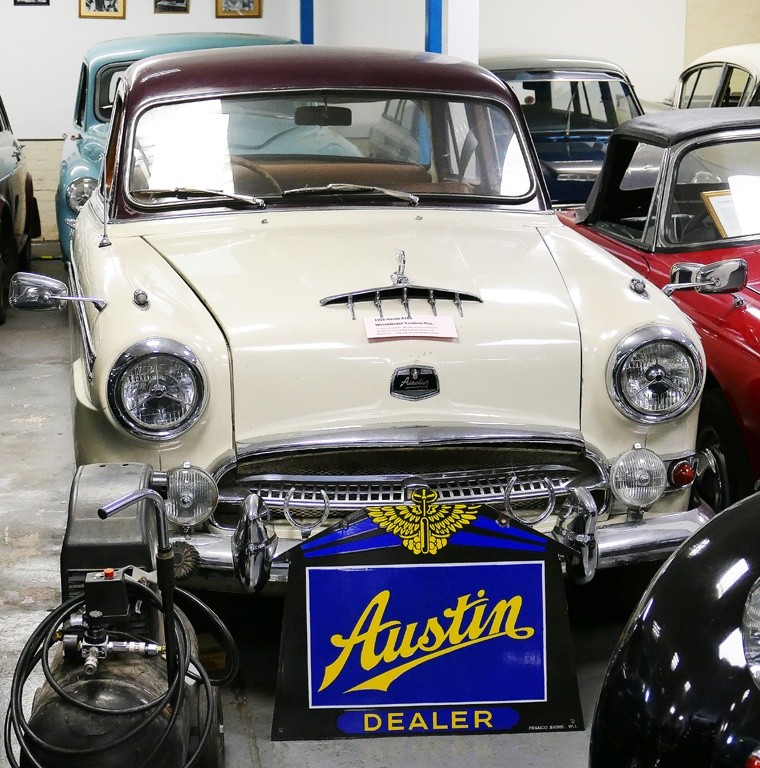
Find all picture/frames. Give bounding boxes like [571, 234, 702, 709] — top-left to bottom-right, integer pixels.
[215, 0, 263, 19]
[79, 0, 125, 20]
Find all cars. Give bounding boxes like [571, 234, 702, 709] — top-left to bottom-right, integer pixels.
[54, 31, 365, 271]
[549, 103, 760, 503]
[656, 41, 760, 112]
[7, 40, 721, 633]
[0, 93, 43, 327]
[470, 53, 724, 226]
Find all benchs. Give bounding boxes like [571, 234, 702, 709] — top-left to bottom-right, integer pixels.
[231, 162, 433, 194]
